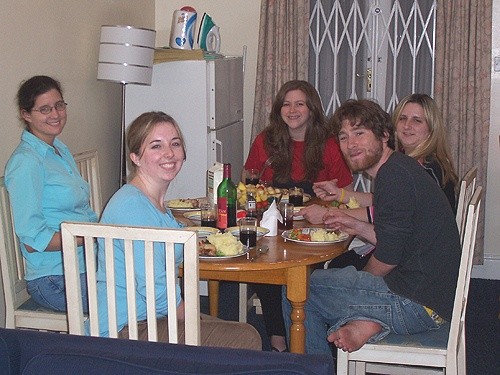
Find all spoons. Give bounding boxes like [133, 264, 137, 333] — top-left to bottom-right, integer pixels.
[317, 186, 336, 196]
[250, 245, 269, 261]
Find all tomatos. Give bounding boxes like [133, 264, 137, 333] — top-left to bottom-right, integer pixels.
[297, 234, 311, 241]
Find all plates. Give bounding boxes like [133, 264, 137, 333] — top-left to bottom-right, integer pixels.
[280, 189, 312, 204]
[257, 196, 280, 207]
[182, 211, 216, 225]
[224, 225, 270, 241]
[281, 228, 350, 246]
[171, 207, 209, 211]
[184, 226, 222, 242]
[293, 206, 308, 220]
[198, 247, 251, 260]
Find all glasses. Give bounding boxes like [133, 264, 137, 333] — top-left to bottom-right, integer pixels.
[27, 102, 67, 114]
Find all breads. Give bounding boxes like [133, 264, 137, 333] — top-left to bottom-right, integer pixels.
[311, 228, 338, 241]
[206, 231, 245, 257]
[168, 200, 194, 207]
[346, 196, 360, 209]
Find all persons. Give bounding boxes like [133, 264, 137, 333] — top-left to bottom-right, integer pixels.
[294, 94, 460, 279]
[241, 80, 354, 354]
[282, 99, 462, 354]
[83, 112, 262, 352]
[4, 75, 99, 314]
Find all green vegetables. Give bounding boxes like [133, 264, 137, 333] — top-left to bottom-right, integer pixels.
[288, 230, 298, 240]
[199, 239, 224, 257]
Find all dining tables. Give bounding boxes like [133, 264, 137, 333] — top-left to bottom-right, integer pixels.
[171, 197, 354, 355]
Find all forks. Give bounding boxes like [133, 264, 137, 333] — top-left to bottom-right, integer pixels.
[261, 156, 276, 179]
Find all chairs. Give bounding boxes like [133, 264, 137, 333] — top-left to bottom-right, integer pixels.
[60, 221, 200, 347]
[336, 167, 482, 375]
[0, 176, 89, 335]
[71, 150, 103, 222]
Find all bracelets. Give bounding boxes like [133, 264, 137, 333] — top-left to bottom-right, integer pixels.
[338, 188, 345, 203]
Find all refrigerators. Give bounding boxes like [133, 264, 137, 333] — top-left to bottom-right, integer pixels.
[123, 52, 244, 202]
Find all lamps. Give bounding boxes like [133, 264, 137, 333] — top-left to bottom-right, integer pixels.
[96, 24, 156, 188]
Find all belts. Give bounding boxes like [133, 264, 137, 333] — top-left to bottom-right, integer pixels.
[424, 306, 447, 325]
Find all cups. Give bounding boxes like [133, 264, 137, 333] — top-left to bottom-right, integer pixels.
[278, 203, 293, 229]
[288, 188, 304, 205]
[201, 210, 217, 227]
[245, 169, 259, 185]
[239, 216, 258, 249]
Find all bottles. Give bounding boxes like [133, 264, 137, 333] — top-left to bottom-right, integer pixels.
[246, 192, 256, 210]
[217, 163, 237, 233]
